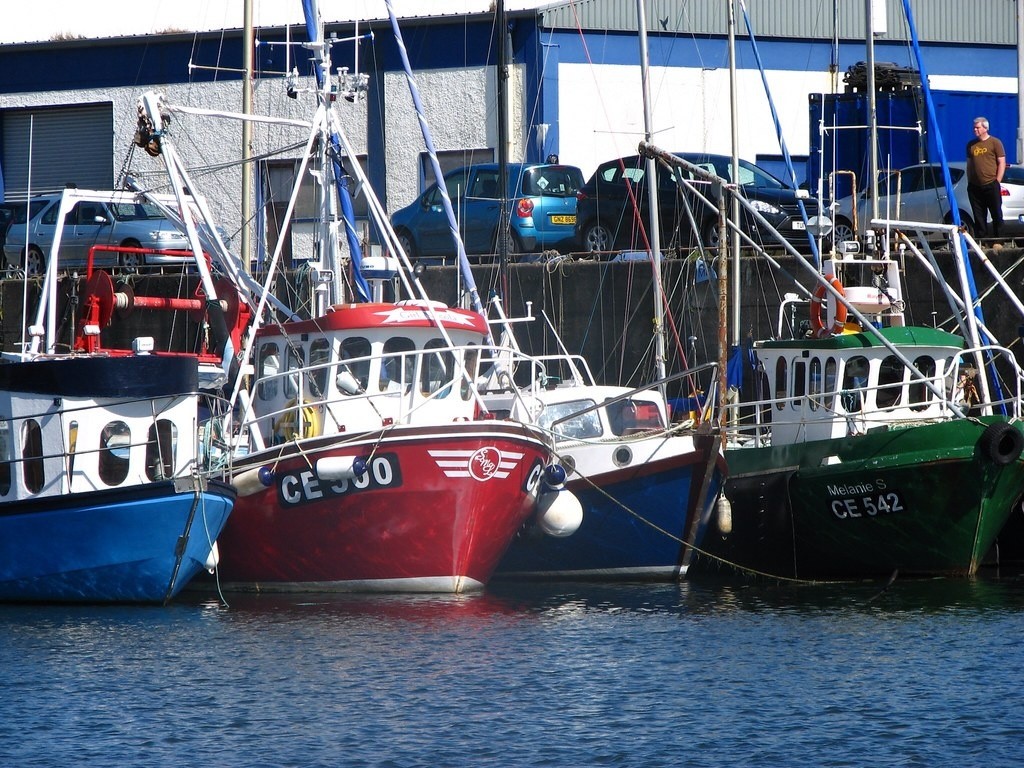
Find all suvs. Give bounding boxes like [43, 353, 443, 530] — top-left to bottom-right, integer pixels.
[3, 190, 231, 274]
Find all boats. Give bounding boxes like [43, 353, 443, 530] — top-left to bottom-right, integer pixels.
[69, 246, 555, 598]
[0, 325, 237, 607]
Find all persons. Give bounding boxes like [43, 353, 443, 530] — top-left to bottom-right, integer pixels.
[966, 117, 1006, 247]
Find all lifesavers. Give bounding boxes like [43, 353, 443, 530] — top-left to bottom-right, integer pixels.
[982, 422, 1024, 468]
[326, 302, 395, 314]
[810, 275, 848, 340]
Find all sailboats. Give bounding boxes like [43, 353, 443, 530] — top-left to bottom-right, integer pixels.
[361, 2, 730, 583]
[670, 0, 1024, 578]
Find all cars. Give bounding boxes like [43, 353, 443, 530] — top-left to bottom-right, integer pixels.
[388, 163, 586, 263]
[827, 161, 1023, 253]
[576, 153, 836, 261]
